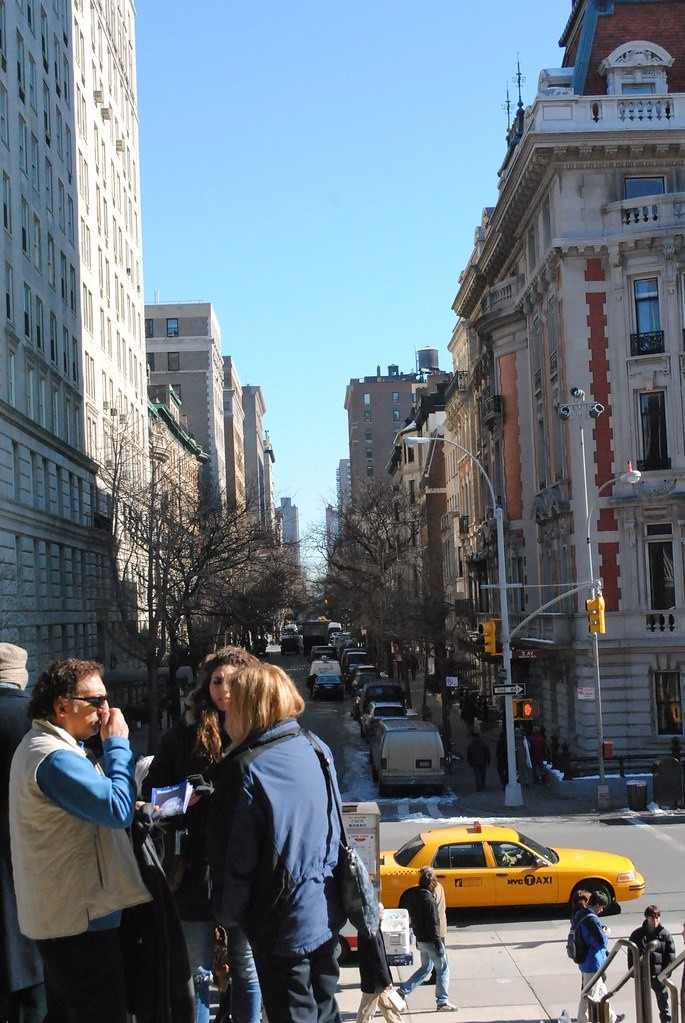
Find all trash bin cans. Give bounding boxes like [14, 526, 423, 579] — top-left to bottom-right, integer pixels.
[626, 780, 647, 811]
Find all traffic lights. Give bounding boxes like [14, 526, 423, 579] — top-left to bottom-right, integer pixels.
[521, 698, 539, 721]
[477, 619, 496, 655]
[585, 594, 606, 634]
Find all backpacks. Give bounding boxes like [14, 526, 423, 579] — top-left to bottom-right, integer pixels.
[566, 911, 599, 963]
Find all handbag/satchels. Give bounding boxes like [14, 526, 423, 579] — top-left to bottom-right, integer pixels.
[331, 843, 381, 936]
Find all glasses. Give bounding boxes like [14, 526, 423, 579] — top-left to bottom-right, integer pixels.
[57, 692, 109, 709]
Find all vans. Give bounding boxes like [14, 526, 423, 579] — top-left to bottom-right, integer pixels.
[366, 719, 448, 795]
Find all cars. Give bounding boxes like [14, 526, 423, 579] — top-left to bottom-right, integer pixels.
[312, 673, 346, 702]
[280, 614, 388, 697]
[380, 821, 646, 918]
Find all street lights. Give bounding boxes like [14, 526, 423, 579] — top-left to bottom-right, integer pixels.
[404, 435, 523, 807]
[585, 470, 643, 808]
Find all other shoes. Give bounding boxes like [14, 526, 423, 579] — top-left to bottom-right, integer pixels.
[212, 984, 232, 1023]
[660, 1014, 671, 1021]
[615, 1014, 626, 1023]
[423, 977, 437, 985]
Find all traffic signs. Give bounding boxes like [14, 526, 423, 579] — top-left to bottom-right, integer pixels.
[492, 683, 527, 696]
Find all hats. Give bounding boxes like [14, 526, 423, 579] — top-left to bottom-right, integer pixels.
[0, 642, 30, 691]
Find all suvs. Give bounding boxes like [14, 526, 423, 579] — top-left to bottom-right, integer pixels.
[359, 701, 407, 740]
[351, 681, 408, 721]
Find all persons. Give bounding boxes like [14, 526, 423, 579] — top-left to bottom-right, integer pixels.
[356, 903, 402, 1023]
[571, 889, 625, 1023]
[467, 733, 491, 792]
[1, 641, 47, 1023]
[209, 663, 344, 1023]
[450, 689, 476, 736]
[497, 726, 546, 788]
[493, 843, 522, 868]
[139, 643, 262, 1023]
[397, 865, 459, 1011]
[10, 658, 159, 1023]
[680, 923, 685, 1023]
[628, 905, 676, 1023]
[396, 650, 419, 680]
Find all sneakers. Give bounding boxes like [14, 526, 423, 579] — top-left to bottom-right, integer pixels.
[436, 1002, 458, 1012]
